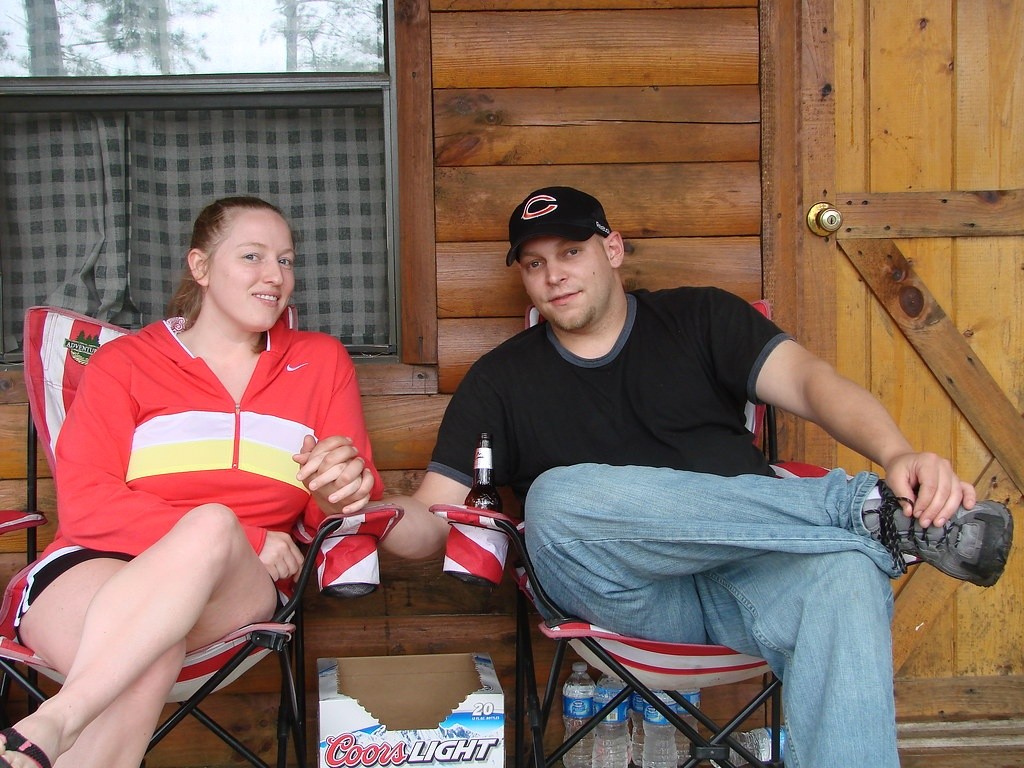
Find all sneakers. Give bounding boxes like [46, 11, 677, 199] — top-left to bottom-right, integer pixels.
[862, 476, 1014, 589]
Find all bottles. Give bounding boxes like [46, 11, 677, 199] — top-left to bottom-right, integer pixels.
[676, 688, 700, 767]
[710, 725, 787, 768]
[463, 434, 503, 512]
[645, 688, 679, 768]
[631, 685, 644, 766]
[562, 660, 596, 768]
[593, 672, 633, 768]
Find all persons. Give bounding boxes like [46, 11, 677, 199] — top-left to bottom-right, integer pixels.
[299, 187, 1012, 768]
[0, 198, 384, 768]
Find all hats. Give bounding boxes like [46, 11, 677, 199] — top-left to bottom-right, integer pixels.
[506, 186, 612, 266]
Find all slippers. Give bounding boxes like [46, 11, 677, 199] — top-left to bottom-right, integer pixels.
[0, 725, 51, 768]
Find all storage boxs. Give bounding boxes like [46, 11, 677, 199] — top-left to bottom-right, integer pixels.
[316, 651, 506, 768]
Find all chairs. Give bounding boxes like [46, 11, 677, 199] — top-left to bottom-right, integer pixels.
[428, 298, 923, 768]
[0, 302, 407, 768]
